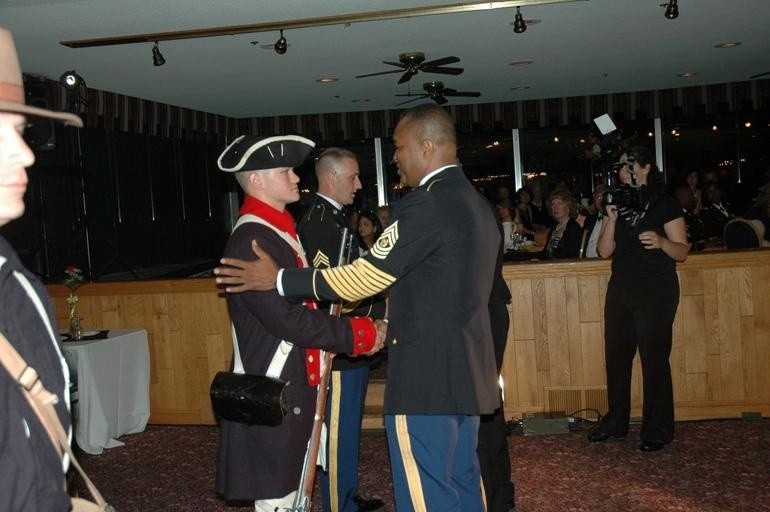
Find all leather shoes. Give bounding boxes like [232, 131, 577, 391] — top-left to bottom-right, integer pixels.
[585, 414, 627, 444]
[639, 433, 676, 454]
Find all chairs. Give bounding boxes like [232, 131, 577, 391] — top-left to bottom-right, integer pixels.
[501, 208, 763, 263]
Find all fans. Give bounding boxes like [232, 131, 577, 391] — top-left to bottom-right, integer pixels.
[393, 80, 482, 106]
[354, 51, 465, 85]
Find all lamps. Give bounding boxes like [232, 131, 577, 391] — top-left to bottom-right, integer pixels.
[150, 1, 682, 69]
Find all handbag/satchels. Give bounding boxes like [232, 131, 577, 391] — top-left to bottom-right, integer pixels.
[209, 369, 288, 427]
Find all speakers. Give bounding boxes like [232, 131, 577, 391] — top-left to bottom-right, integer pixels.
[24, 82, 57, 151]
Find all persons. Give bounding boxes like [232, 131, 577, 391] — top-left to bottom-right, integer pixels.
[458, 149, 517, 512]
[0, 23, 117, 512]
[207, 100, 501, 510]
[347, 206, 391, 250]
[211, 129, 389, 512]
[494, 179, 608, 262]
[294, 145, 389, 511]
[678, 167, 770, 252]
[585, 139, 689, 453]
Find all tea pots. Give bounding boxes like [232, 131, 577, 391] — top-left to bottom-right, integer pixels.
[502, 222, 517, 249]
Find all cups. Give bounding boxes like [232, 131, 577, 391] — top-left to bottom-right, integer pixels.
[70, 317, 82, 343]
[510, 232, 532, 245]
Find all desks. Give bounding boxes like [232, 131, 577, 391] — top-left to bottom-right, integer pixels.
[57, 327, 154, 456]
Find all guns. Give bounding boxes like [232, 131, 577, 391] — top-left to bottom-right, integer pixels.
[286, 228, 353, 512]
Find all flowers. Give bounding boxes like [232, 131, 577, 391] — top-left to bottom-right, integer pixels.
[62, 262, 86, 317]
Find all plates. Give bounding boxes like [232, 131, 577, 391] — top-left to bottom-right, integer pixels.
[80, 331, 100, 337]
[60, 335, 69, 341]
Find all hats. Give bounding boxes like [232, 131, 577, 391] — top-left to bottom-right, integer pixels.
[217, 133, 317, 173]
[0, 26, 83, 127]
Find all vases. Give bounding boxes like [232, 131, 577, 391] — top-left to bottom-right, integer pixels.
[66, 302, 84, 340]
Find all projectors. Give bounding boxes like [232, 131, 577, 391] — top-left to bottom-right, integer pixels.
[59, 70, 90, 116]
